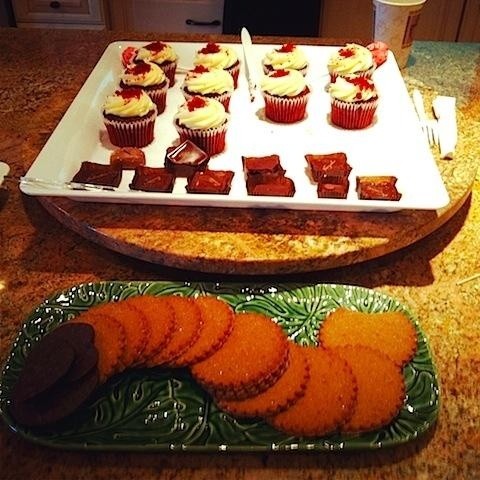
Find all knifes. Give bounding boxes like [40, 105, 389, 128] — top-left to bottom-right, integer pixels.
[238, 26, 263, 99]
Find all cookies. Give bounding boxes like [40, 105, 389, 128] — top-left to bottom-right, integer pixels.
[11, 294, 418, 438]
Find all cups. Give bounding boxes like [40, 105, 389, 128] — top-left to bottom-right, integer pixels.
[372, 1, 428, 68]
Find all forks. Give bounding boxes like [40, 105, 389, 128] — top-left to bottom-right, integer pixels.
[412, 85, 439, 148]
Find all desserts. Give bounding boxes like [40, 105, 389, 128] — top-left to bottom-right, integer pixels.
[69, 144, 403, 201]
[99, 40, 241, 149]
[327, 43, 378, 128]
[260, 40, 309, 123]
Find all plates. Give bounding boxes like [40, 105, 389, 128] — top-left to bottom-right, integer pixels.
[0, 279, 440, 457]
[20, 39, 450, 215]
